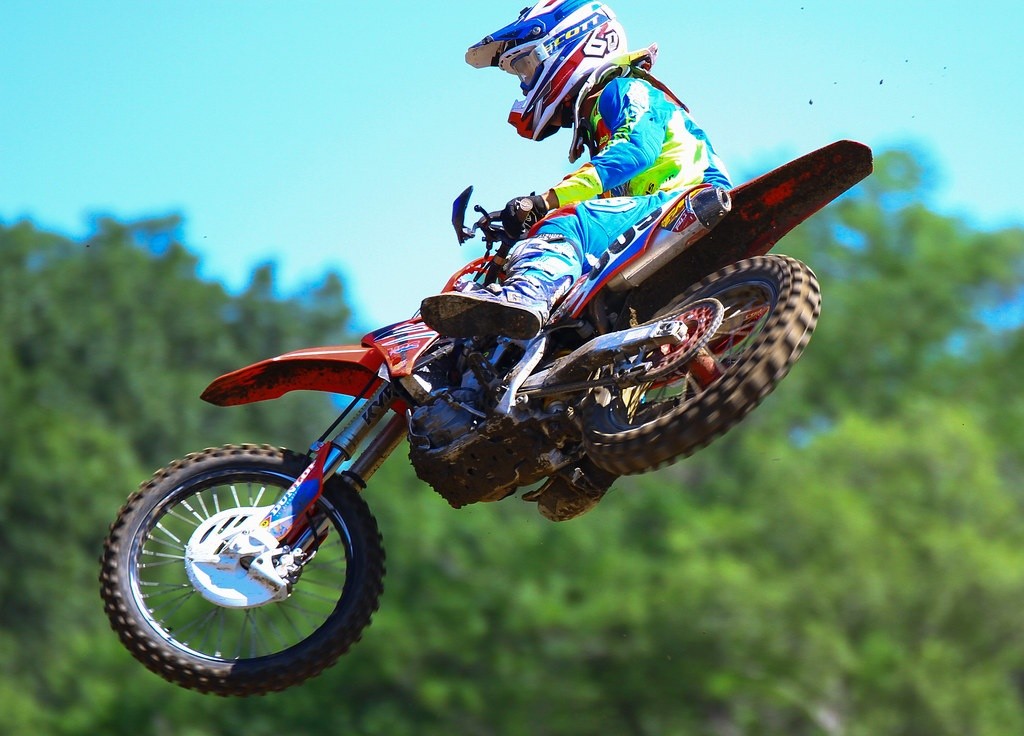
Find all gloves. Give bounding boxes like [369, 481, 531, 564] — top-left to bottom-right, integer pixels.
[502, 191, 547, 238]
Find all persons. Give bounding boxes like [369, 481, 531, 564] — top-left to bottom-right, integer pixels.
[418, 0, 733, 524]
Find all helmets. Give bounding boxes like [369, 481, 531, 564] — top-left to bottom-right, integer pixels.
[465, 0, 627, 141]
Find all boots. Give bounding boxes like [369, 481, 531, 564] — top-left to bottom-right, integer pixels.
[538, 456, 621, 523]
[420, 237, 582, 340]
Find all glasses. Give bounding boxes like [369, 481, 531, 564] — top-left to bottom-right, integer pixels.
[510, 44, 547, 92]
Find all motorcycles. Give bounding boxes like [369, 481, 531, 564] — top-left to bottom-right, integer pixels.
[97, 140, 873, 699]
